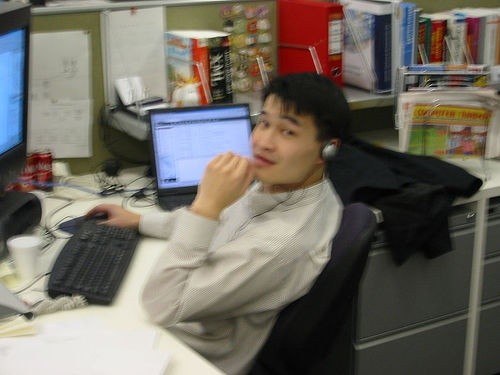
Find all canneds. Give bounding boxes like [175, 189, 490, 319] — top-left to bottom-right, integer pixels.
[16, 150, 53, 194]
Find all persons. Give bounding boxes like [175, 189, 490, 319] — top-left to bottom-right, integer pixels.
[85, 73, 349, 375]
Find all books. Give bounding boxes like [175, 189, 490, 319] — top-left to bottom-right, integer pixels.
[344, 0, 500, 170]
[169, 28, 233, 108]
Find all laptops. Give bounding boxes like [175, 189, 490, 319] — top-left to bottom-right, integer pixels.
[150, 103, 255, 210]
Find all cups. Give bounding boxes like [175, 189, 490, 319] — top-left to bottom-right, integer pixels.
[7, 234, 42, 278]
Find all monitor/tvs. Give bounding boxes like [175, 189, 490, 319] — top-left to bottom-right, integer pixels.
[0, 0, 34, 201]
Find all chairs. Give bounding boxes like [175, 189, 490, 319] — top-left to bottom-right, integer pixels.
[244, 202, 375, 375]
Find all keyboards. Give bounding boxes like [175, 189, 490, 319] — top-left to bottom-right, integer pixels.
[46, 215, 139, 306]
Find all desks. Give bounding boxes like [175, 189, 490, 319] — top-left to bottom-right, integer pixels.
[439, 158, 500, 375]
